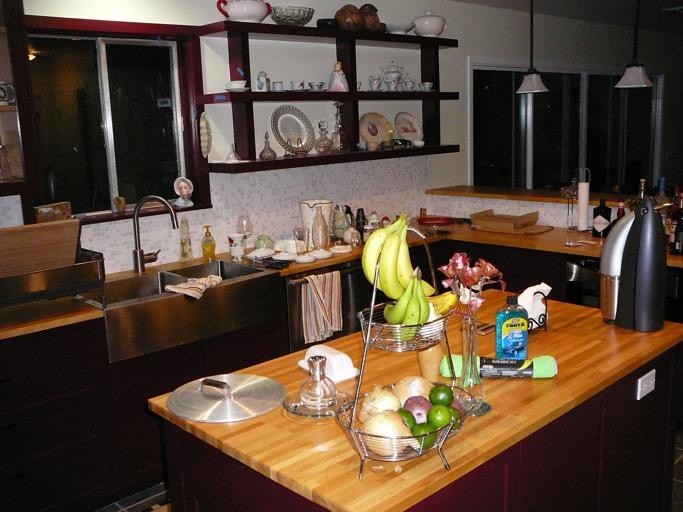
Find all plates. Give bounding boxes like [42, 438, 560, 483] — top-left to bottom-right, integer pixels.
[359, 111, 425, 151]
[416, 217, 456, 227]
[271, 105, 316, 153]
[384, 22, 416, 35]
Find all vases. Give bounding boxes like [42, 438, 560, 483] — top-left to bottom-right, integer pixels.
[450, 313, 486, 415]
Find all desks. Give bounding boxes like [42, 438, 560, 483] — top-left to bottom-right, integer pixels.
[145, 286, 681, 511]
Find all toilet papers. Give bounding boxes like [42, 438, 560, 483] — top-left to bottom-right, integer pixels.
[577, 182, 591, 231]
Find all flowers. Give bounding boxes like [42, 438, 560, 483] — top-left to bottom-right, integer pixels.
[436, 248, 507, 384]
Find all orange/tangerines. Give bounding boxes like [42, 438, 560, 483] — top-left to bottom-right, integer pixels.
[428, 405, 452, 428]
[428, 384, 455, 406]
[447, 406, 462, 429]
[410, 424, 437, 449]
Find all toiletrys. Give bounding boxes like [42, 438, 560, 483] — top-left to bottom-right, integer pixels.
[200, 225, 217, 261]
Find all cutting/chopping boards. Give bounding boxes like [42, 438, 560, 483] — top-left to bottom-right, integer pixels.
[0, 217, 80, 283]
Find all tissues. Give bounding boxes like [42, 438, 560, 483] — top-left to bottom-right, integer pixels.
[506, 283, 553, 330]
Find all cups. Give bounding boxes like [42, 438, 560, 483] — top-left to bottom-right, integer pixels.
[225, 205, 383, 266]
[368, 61, 436, 92]
[224, 70, 328, 93]
[0, 84, 17, 106]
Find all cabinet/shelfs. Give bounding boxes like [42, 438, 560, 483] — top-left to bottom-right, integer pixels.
[0, 0, 43, 202]
[196, 14, 467, 178]
[0, 315, 123, 511]
[441, 240, 567, 307]
[103, 316, 287, 502]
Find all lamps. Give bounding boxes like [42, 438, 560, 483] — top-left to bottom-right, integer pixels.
[512, 1, 551, 96]
[612, 1, 656, 92]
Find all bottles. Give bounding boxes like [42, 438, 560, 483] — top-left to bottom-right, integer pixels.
[259, 129, 277, 160]
[316, 102, 347, 155]
[0, 135, 14, 184]
[577, 177, 683, 255]
[299, 354, 336, 410]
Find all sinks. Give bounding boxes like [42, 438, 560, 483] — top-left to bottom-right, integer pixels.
[163, 263, 281, 350]
[100, 271, 203, 364]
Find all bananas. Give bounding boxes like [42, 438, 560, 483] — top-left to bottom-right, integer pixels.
[430, 290, 458, 314]
[383, 265, 431, 343]
[362, 214, 435, 300]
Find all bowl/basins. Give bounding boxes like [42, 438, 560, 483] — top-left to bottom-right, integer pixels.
[318, 17, 337, 29]
[271, 7, 316, 26]
[415, 11, 446, 38]
[215, 0, 273, 24]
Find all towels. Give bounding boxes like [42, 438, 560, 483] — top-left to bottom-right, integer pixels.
[298, 270, 346, 347]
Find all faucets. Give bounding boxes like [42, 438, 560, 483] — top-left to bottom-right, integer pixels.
[131, 194, 180, 275]
[47, 172, 58, 204]
[91, 187, 101, 208]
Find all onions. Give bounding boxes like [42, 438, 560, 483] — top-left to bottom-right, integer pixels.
[362, 409, 420, 457]
[343, 227, 360, 244]
[356, 390, 402, 424]
[393, 375, 434, 408]
